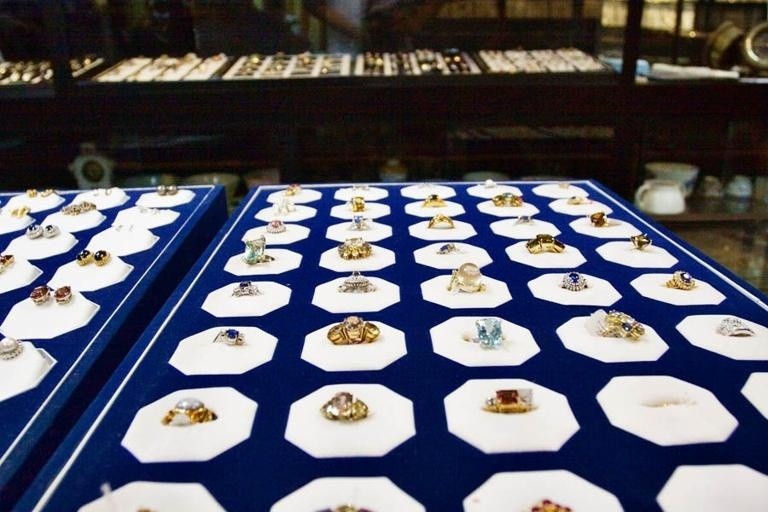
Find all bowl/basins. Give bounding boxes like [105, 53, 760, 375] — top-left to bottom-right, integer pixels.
[183, 173, 239, 204]
[641, 161, 700, 196]
[124, 174, 179, 188]
[724, 174, 752, 200]
[462, 170, 509, 181]
[697, 175, 723, 200]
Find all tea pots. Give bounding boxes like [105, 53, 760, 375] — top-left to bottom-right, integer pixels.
[634, 178, 686, 215]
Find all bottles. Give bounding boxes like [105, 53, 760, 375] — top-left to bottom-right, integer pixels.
[379, 156, 407, 182]
[68, 141, 112, 189]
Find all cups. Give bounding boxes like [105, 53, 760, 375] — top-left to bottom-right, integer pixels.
[243, 167, 280, 190]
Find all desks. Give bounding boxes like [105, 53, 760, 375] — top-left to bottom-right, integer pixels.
[0, 45, 768, 246]
